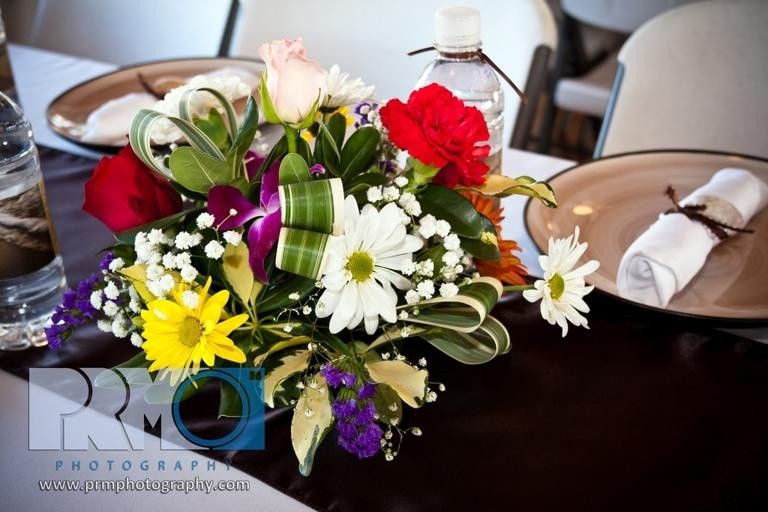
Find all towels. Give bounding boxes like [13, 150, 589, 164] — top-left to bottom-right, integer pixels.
[617, 165, 767, 308]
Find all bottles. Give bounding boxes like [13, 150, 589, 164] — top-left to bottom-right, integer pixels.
[0, 91, 76, 353]
[412, 8, 505, 226]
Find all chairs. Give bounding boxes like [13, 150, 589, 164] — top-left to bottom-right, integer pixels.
[215, 1, 768, 174]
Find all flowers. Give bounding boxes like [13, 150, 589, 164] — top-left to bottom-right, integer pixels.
[43, 37, 604, 479]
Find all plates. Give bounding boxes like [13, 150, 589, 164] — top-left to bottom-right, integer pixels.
[47, 56, 267, 152]
[523, 149, 768, 328]
